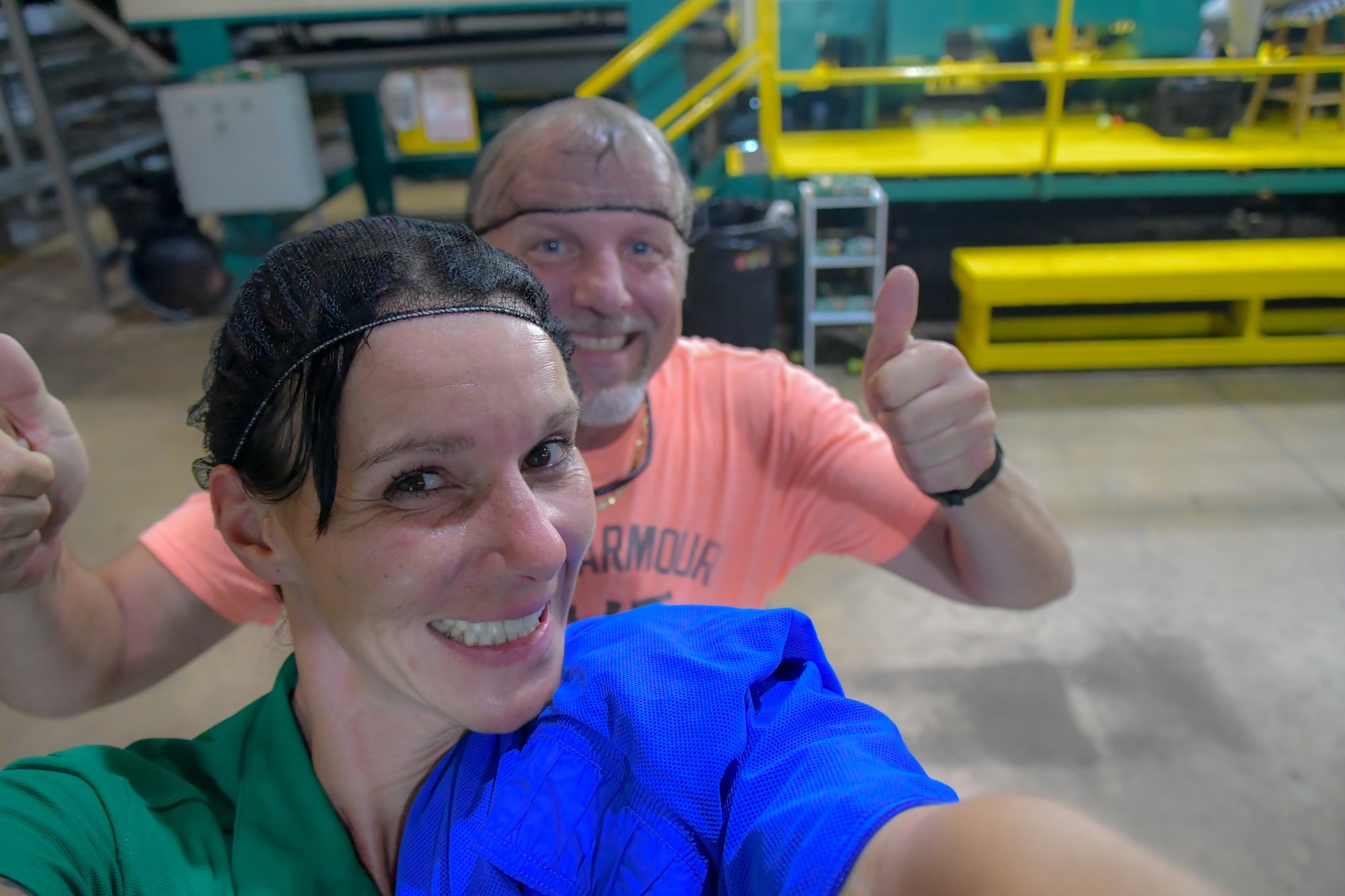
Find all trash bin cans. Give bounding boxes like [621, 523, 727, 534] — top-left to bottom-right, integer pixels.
[677, 198, 799, 347]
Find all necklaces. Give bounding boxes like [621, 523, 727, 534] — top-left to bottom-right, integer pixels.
[585, 404, 651, 514]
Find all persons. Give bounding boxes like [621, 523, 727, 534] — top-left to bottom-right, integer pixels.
[2, 93, 1076, 735]
[1, 213, 1233, 896]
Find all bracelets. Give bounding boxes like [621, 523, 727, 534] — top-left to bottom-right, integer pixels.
[922, 437, 1009, 510]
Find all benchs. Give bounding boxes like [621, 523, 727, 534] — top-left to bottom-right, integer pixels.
[954, 238, 1345, 377]
